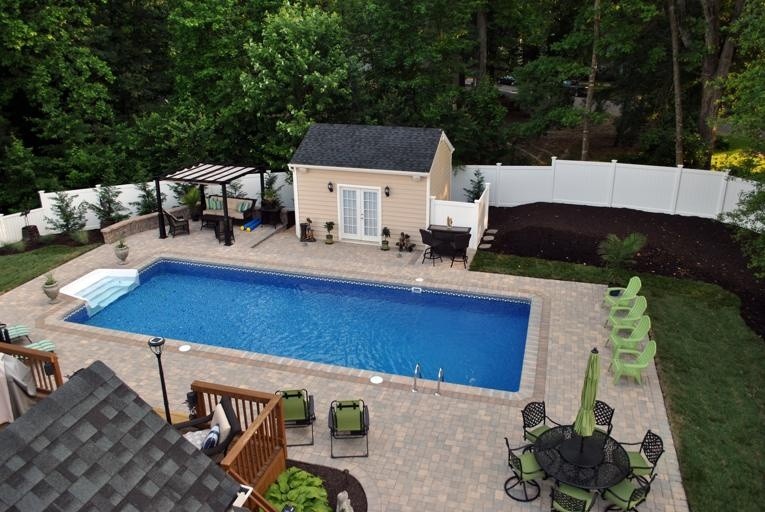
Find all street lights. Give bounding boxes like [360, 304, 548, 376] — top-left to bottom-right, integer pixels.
[148, 336, 172, 425]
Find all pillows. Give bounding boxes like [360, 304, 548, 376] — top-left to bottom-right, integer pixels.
[203, 424, 220, 449]
[206, 198, 250, 211]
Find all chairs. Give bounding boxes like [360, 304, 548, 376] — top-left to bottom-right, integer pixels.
[173, 396, 242, 457]
[328, 400, 370, 458]
[504, 400, 665, 512]
[12, 339, 56, 364]
[602, 276, 658, 385]
[215, 217, 236, 244]
[450, 234, 471, 269]
[163, 208, 189, 238]
[276, 389, 316, 447]
[7, 325, 33, 344]
[419, 229, 443, 267]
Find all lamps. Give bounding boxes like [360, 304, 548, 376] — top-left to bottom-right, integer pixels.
[385, 186, 390, 196]
[328, 181, 333, 192]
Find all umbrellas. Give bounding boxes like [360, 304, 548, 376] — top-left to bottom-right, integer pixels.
[571, 345, 602, 454]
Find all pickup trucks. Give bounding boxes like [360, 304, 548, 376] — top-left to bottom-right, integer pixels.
[564, 80, 588, 97]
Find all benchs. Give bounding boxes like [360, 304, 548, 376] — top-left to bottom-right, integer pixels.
[203, 195, 257, 225]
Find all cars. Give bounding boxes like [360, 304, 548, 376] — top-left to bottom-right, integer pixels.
[497, 75, 515, 86]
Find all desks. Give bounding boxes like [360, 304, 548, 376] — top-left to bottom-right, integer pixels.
[428, 225, 471, 249]
[201, 214, 222, 231]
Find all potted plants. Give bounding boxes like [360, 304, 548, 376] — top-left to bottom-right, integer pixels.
[381, 227, 390, 250]
[259, 171, 285, 229]
[43, 271, 60, 304]
[323, 222, 334, 244]
[597, 232, 648, 296]
[182, 187, 201, 221]
[114, 230, 130, 265]
